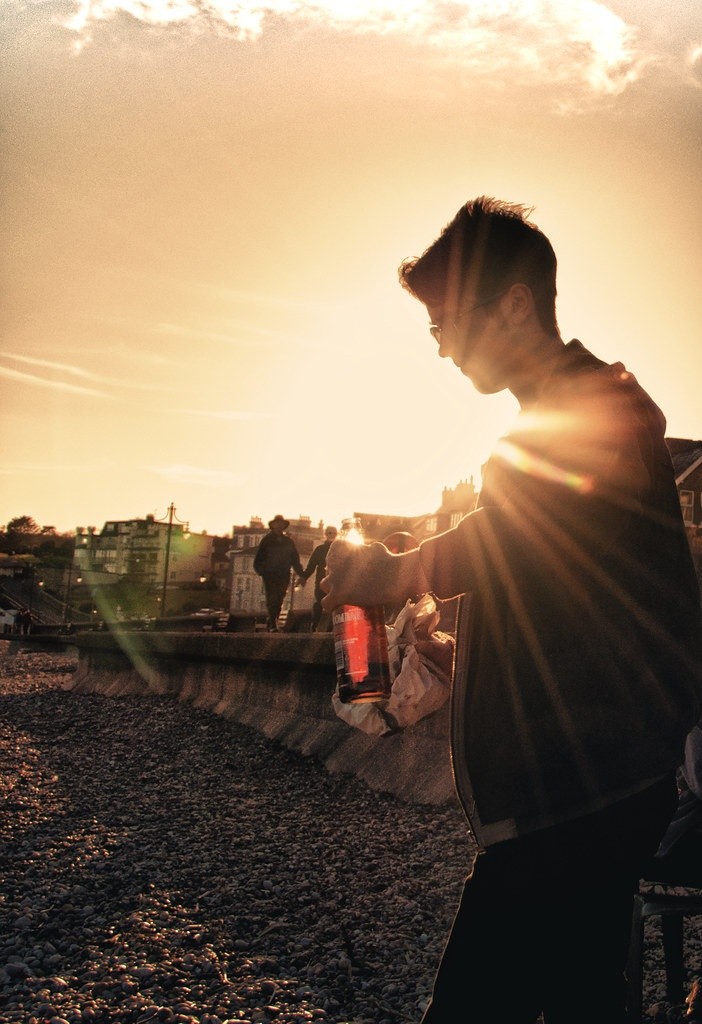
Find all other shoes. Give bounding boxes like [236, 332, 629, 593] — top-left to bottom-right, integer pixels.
[310, 623, 317, 631]
[269, 623, 278, 632]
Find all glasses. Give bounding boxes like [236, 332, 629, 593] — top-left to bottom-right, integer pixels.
[429, 286, 511, 346]
[328, 532, 337, 535]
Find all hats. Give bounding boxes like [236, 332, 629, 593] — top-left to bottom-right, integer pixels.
[268, 515, 289, 531]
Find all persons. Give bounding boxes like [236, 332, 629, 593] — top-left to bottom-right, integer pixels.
[254, 516, 306, 634]
[319, 197, 702, 1024]
[297, 527, 340, 632]
[14, 608, 32, 636]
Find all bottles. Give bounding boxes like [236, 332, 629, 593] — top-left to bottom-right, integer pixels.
[331, 604, 394, 703]
